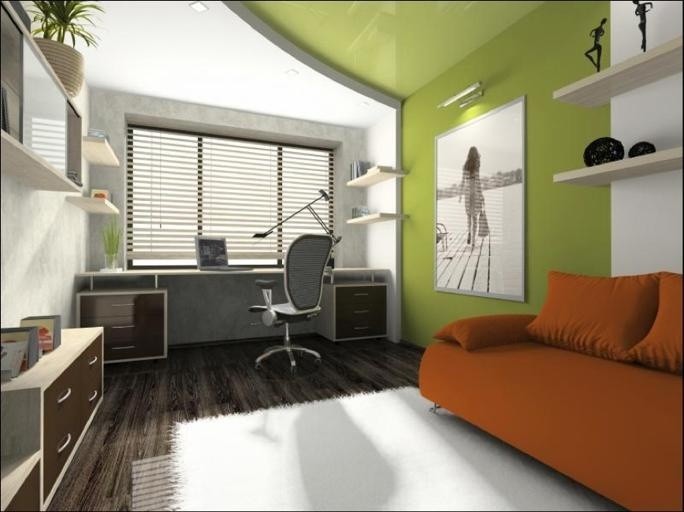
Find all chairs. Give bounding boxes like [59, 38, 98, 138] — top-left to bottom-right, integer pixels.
[249, 234, 333, 378]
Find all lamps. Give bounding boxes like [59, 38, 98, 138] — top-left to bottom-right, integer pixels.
[438, 80, 484, 111]
[251, 188, 343, 265]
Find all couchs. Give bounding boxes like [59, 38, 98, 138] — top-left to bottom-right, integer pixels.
[419, 272, 684, 512]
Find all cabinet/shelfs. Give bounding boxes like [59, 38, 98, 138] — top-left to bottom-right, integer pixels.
[66, 138, 121, 215]
[553, 34, 683, 186]
[346, 167, 409, 224]
[1, 326, 106, 512]
[0, 0, 87, 194]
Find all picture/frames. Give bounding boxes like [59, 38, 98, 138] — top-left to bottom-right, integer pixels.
[195, 235, 228, 270]
[434, 94, 527, 302]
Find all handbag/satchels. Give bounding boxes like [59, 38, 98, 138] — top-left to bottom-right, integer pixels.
[479, 213, 489, 237]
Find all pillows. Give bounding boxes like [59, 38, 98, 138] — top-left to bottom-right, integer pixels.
[628, 271, 684, 376]
[526, 271, 660, 365]
[433, 313, 538, 351]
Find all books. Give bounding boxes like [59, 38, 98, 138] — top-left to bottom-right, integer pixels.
[1, 341, 28, 378]
[352, 207, 367, 218]
[351, 160, 393, 180]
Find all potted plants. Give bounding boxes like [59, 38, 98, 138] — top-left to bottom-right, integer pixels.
[26, 0, 108, 101]
[101, 215, 123, 272]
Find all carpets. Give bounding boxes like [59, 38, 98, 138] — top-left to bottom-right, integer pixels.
[163, 386, 630, 512]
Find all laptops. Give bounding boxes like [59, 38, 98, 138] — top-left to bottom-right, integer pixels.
[195, 234, 253, 271]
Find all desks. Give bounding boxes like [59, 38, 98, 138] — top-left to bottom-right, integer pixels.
[76, 268, 388, 366]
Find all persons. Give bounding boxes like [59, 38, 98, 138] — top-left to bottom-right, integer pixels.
[584, 17, 608, 72]
[632, 0, 653, 53]
[458, 146, 485, 247]
